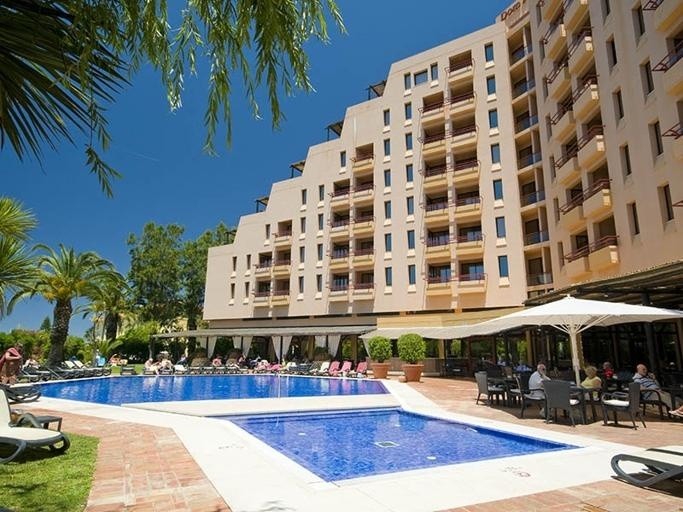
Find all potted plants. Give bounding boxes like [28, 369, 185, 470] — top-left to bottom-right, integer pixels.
[368, 334, 393, 381]
[397, 334, 426, 382]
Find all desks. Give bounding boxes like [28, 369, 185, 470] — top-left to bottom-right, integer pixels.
[31, 414, 63, 431]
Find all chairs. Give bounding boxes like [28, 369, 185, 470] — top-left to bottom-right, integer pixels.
[0, 384, 40, 403]
[474, 362, 683, 430]
[15, 356, 136, 383]
[0, 390, 64, 464]
[447, 355, 467, 376]
[609, 445, 682, 488]
[142, 356, 367, 378]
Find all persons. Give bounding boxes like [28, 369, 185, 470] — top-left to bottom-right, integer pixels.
[0, 340, 26, 384]
[94, 349, 120, 367]
[632, 364, 682, 413]
[145, 353, 187, 375]
[526, 363, 551, 418]
[211, 347, 267, 375]
[602, 362, 615, 378]
[667, 406, 682, 417]
[578, 365, 601, 402]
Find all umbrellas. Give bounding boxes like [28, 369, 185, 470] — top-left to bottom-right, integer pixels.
[466, 293, 682, 385]
